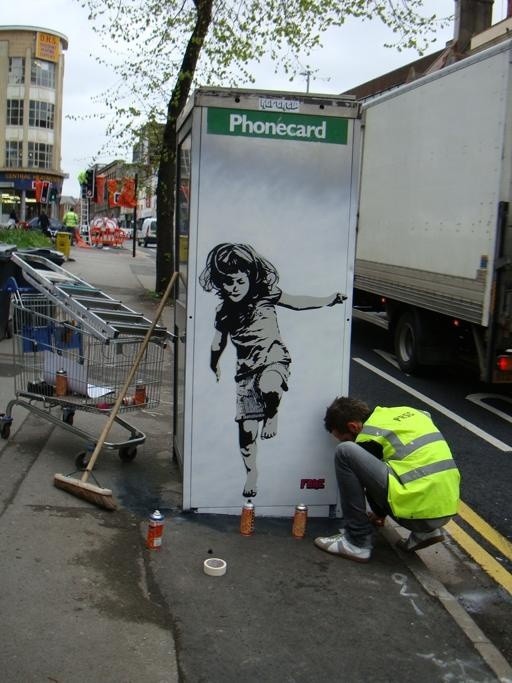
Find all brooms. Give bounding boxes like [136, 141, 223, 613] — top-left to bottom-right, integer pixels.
[54, 271, 181, 512]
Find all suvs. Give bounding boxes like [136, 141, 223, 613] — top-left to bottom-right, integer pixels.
[136, 218, 158, 250]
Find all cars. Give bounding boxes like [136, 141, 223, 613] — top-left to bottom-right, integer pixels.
[26, 217, 65, 243]
[88, 218, 126, 247]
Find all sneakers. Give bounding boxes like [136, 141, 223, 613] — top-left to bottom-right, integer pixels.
[314, 529, 371, 563]
[396, 528, 446, 553]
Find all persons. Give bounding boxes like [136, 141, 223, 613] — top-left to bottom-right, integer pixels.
[10, 207, 20, 222]
[38, 210, 51, 232]
[311, 395, 460, 561]
[63, 206, 80, 246]
[201, 237, 350, 501]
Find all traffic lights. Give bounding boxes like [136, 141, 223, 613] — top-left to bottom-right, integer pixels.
[35, 180, 50, 203]
[82, 167, 95, 200]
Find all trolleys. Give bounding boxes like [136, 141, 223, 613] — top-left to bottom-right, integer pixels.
[0, 276, 167, 470]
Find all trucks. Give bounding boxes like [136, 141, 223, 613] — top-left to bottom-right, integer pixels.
[353, 37, 512, 385]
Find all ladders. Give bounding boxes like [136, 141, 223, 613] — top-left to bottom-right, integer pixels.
[11, 253, 178, 349]
[80, 186, 91, 246]
